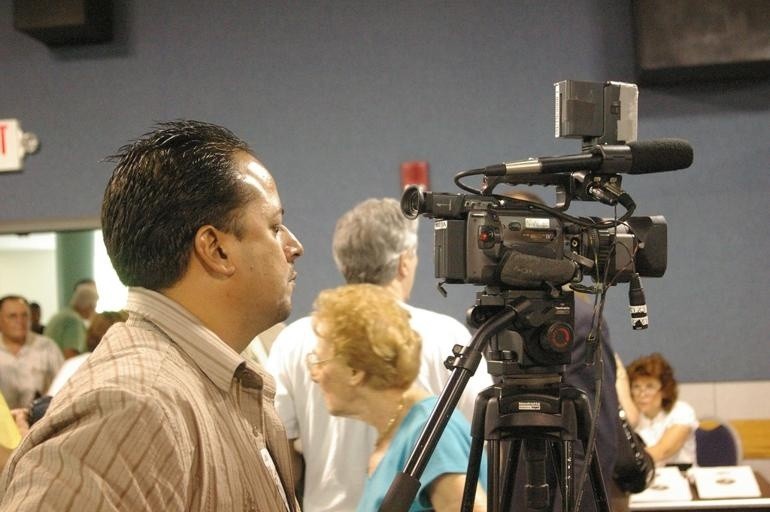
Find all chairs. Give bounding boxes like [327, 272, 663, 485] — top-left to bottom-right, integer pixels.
[695, 414, 744, 466]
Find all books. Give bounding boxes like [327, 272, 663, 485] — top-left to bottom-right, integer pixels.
[631, 466, 692, 502]
[690, 466, 764, 500]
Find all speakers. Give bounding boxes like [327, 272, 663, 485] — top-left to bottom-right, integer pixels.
[12, 0, 113, 48]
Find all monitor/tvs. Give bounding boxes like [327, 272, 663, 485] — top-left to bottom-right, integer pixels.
[632, 0, 770, 86]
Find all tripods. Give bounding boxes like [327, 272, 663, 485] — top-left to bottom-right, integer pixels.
[380, 293, 611, 511]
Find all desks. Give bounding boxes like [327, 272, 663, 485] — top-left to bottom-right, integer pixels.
[630, 467, 770, 511]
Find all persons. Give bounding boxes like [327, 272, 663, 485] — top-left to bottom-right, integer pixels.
[0, 117, 305, 511]
[486, 190, 622, 512]
[0, 279, 129, 477]
[266, 194, 497, 512]
[305, 280, 489, 512]
[625, 352, 701, 467]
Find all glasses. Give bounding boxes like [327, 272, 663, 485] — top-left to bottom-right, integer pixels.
[304, 347, 351, 378]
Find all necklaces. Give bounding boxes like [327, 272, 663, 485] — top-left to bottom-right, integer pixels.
[373, 395, 406, 447]
